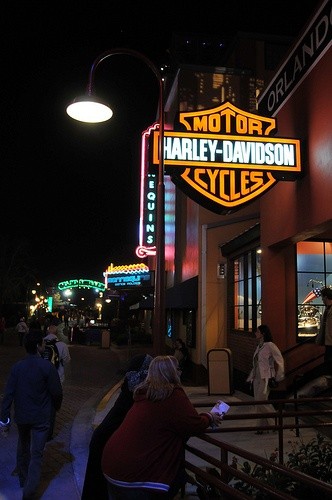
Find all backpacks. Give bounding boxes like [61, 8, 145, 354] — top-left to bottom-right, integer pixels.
[42, 339, 62, 368]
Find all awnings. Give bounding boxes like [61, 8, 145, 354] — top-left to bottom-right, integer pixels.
[165, 275, 198, 309]
[139, 297, 154, 311]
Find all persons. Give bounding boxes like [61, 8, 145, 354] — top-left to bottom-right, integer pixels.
[81, 354, 154, 500]
[317, 288, 332, 395]
[44, 326, 71, 387]
[16, 318, 28, 347]
[29, 318, 41, 336]
[0, 333, 62, 500]
[102, 356, 223, 500]
[246, 325, 285, 436]
[0, 317, 6, 335]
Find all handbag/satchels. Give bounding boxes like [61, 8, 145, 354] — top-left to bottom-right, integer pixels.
[269, 377, 278, 388]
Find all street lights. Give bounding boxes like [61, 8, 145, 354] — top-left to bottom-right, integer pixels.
[66, 50, 165, 357]
[27, 280, 41, 318]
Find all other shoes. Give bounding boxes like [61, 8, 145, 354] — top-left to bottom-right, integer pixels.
[256, 430, 271, 435]
